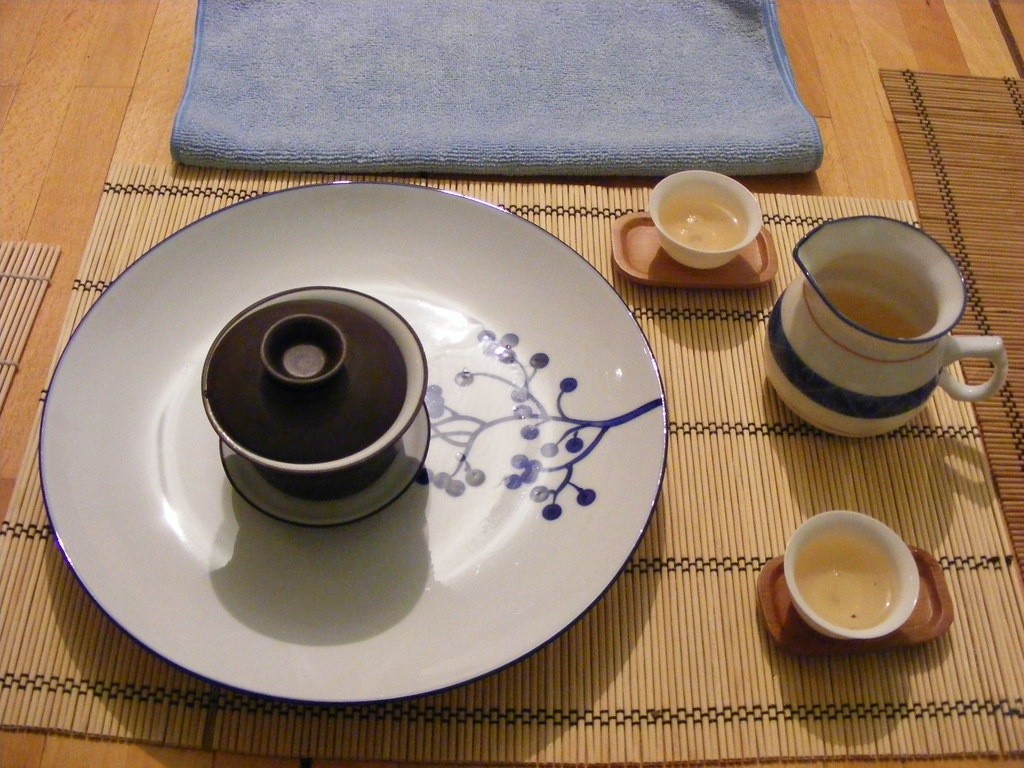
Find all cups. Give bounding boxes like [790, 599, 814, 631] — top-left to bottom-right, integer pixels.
[648, 170, 762, 270]
[202, 287, 428, 501]
[784, 510, 919, 639]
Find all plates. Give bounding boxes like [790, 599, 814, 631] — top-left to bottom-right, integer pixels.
[38, 181, 669, 702]
[216, 400, 432, 526]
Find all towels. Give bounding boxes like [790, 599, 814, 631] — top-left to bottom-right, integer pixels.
[165, 0, 828, 183]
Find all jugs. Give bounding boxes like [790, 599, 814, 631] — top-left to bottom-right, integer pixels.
[767, 216, 1006, 438]
